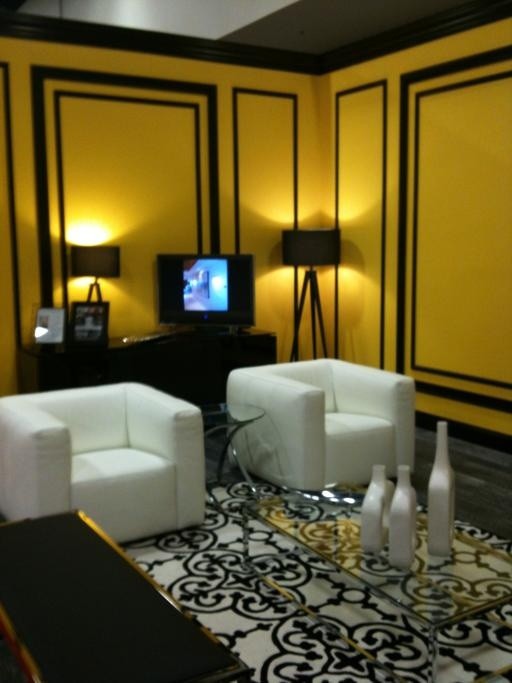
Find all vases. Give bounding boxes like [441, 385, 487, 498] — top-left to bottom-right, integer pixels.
[389, 465, 418, 569]
[359, 465, 394, 549]
[427, 421, 457, 558]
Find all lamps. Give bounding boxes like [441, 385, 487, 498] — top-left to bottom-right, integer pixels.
[282, 227, 340, 361]
[71, 246, 120, 302]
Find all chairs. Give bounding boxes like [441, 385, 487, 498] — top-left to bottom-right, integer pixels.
[226, 357, 416, 493]
[0, 382, 207, 543]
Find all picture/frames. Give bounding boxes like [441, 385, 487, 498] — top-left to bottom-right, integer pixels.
[71, 303, 110, 343]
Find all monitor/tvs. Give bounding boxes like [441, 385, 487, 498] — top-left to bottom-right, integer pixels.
[157, 253, 255, 327]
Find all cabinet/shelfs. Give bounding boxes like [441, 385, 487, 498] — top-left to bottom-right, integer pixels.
[17, 330, 277, 402]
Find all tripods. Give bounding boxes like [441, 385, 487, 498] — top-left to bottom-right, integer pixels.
[289, 270, 328, 362]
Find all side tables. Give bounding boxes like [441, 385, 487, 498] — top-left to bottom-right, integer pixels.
[198, 402, 265, 495]
